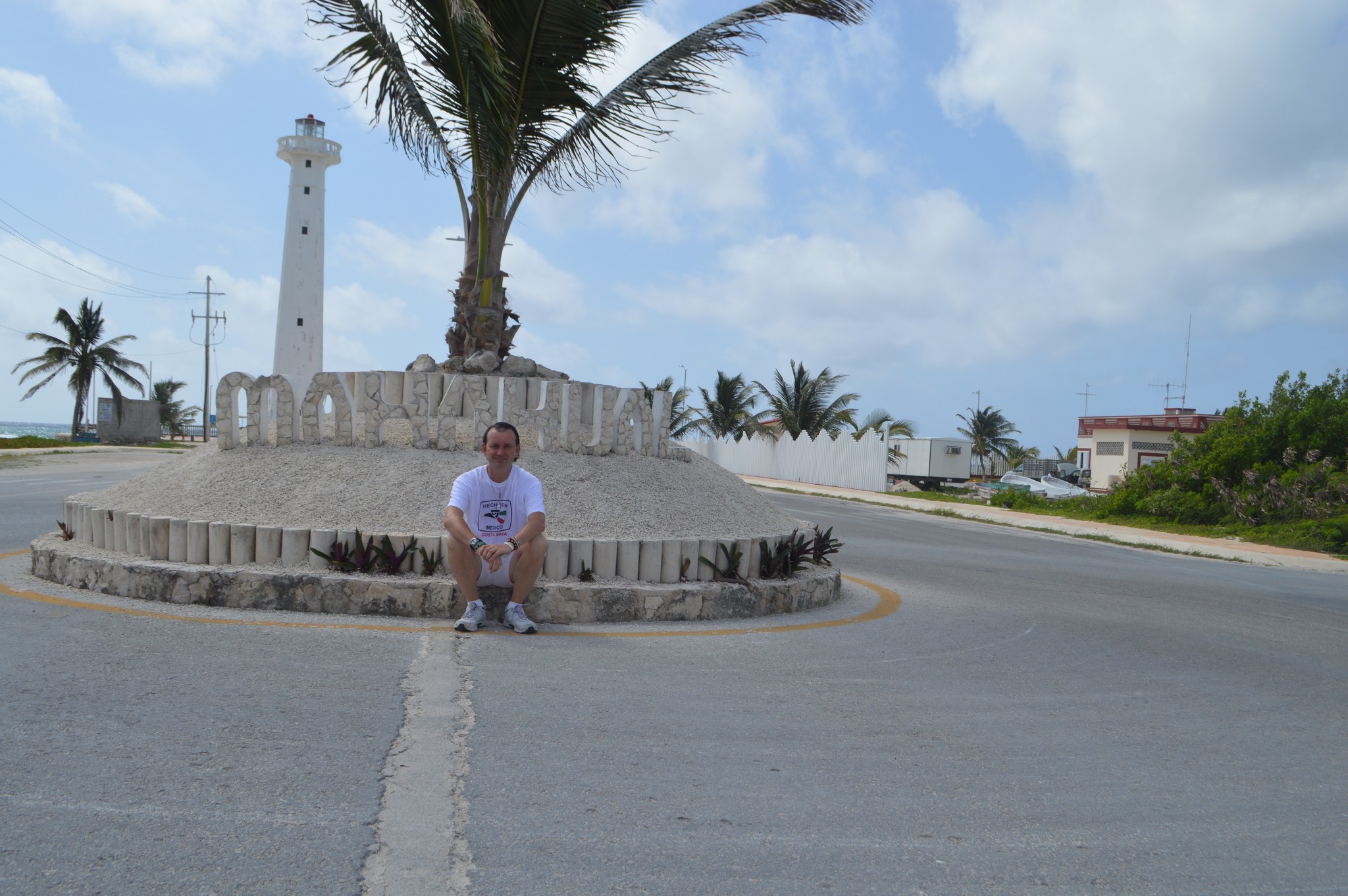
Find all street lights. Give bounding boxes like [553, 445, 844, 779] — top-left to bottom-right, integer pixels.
[972, 390, 980, 418]
[679, 364, 686, 442]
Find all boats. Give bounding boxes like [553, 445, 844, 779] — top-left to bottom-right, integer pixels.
[1041, 475, 1105, 499]
[1000, 474, 1087, 501]
[973, 481, 1047, 503]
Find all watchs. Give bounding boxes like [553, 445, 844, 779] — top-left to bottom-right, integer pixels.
[470, 537, 483, 551]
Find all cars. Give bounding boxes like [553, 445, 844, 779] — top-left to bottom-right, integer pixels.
[1005, 464, 1023, 477]
[1053, 463, 1091, 489]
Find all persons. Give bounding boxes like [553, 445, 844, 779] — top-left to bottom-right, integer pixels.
[443, 422, 546, 634]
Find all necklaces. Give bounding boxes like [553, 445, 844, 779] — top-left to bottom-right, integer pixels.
[486, 465, 510, 510]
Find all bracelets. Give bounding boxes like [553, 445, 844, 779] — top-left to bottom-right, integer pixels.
[475, 542, 486, 552]
[503, 537, 520, 552]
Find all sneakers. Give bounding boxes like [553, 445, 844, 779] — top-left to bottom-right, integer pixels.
[503, 603, 538, 633]
[453, 603, 487, 632]
